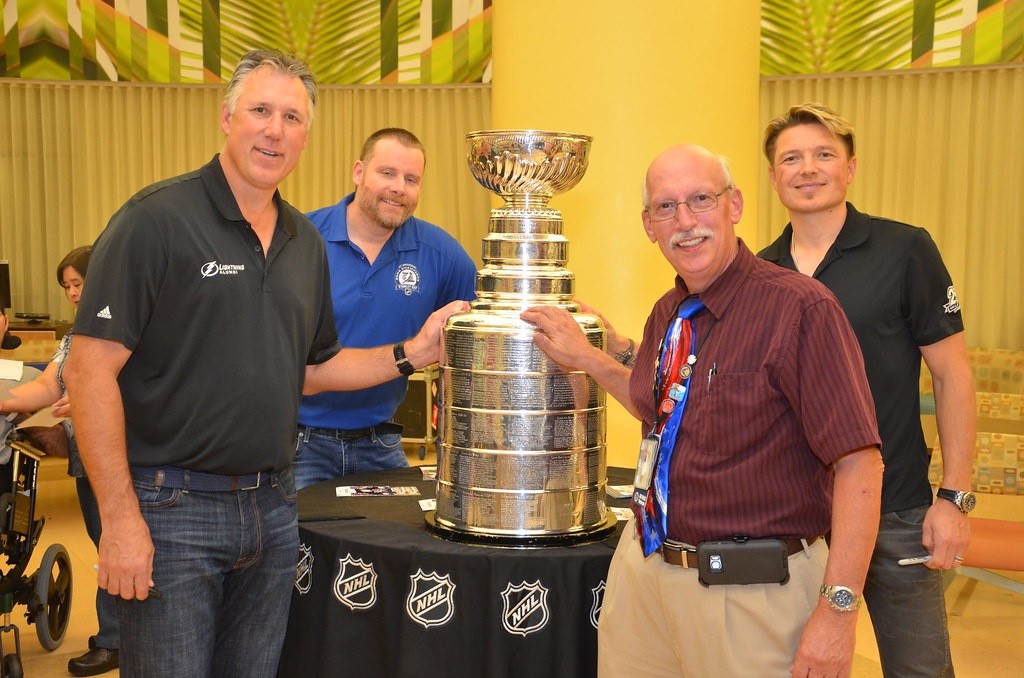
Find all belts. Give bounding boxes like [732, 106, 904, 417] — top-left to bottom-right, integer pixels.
[652, 536, 818, 568]
[298, 419, 406, 440]
[129, 464, 268, 492]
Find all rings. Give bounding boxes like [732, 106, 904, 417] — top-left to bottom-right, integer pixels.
[954, 556, 964, 563]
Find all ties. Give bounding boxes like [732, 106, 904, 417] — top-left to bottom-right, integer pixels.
[642, 298, 703, 559]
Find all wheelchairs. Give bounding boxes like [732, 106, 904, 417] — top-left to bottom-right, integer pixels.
[1, 438, 73, 678]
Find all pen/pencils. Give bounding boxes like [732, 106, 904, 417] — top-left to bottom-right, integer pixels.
[707, 362, 716, 397]
[898, 555, 931, 566]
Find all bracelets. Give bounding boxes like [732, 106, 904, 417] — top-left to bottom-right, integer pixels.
[620, 338, 634, 365]
[393, 341, 415, 377]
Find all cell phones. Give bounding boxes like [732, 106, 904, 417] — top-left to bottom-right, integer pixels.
[696, 540, 788, 585]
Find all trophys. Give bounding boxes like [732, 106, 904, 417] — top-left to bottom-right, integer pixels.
[436, 130, 608, 536]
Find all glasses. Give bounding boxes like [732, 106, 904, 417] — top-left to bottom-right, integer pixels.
[649, 188, 731, 222]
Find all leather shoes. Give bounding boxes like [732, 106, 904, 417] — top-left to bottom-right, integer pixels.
[67, 646, 119, 675]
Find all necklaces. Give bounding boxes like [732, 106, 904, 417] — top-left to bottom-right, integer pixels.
[792, 230, 833, 277]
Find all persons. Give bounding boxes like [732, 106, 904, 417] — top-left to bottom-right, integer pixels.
[520, 143, 885, 678]
[571, 102, 977, 678]
[0, 245, 120, 676]
[292, 127, 479, 491]
[63, 49, 471, 678]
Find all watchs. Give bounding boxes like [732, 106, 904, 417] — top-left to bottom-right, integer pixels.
[820, 584, 862, 612]
[936, 488, 976, 513]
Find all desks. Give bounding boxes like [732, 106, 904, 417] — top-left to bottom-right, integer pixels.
[293, 463, 637, 678]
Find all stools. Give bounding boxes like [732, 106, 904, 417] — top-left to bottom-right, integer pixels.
[942, 514, 1024, 617]
